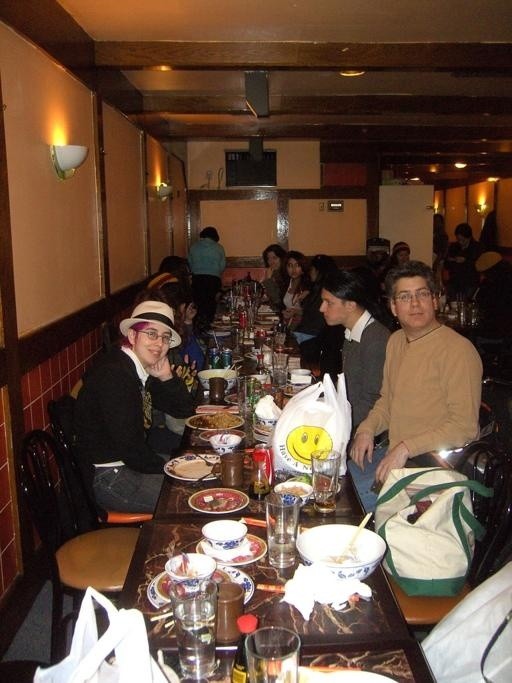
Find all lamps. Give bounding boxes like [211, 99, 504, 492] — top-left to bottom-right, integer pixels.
[156, 181, 173, 198]
[50, 144, 88, 179]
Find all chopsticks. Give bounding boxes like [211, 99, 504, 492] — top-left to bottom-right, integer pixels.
[338, 511, 374, 561]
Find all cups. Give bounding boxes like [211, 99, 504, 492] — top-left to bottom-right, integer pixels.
[248, 470, 271, 500]
[312, 450, 341, 514]
[172, 581, 220, 682]
[220, 453, 244, 487]
[228, 279, 267, 345]
[208, 581, 246, 647]
[246, 626, 301, 683]
[265, 496, 301, 570]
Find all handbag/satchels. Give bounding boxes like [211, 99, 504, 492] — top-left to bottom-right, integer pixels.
[420, 556, 511, 683]
[369, 456, 494, 602]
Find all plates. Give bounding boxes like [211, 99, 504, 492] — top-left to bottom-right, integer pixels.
[196, 532, 267, 566]
[163, 454, 232, 480]
[155, 569, 231, 602]
[184, 290, 322, 439]
[287, 476, 342, 502]
[147, 565, 255, 614]
[188, 488, 250, 514]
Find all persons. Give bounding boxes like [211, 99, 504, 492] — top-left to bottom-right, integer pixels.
[74, 256, 205, 512]
[349, 264, 483, 513]
[433, 214, 510, 306]
[264, 242, 410, 429]
[190, 227, 226, 322]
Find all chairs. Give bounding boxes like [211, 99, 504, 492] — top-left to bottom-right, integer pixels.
[15, 392, 155, 666]
[374, 396, 511, 639]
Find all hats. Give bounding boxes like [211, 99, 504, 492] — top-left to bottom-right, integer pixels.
[117, 297, 184, 351]
[473, 250, 504, 273]
[146, 270, 180, 290]
[391, 241, 411, 256]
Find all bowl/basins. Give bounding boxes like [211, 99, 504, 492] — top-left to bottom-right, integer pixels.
[164, 553, 215, 588]
[297, 522, 387, 584]
[202, 520, 249, 550]
[209, 434, 242, 455]
[273, 481, 312, 509]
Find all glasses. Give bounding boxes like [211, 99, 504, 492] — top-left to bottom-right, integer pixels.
[392, 290, 434, 303]
[134, 326, 177, 344]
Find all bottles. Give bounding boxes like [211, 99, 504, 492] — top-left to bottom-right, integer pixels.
[233, 615, 257, 683]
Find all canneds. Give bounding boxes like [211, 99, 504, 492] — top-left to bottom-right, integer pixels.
[221, 349, 233, 367]
[252, 444, 275, 487]
[207, 347, 220, 369]
[222, 282, 253, 312]
[240, 312, 247, 328]
[257, 329, 267, 338]
